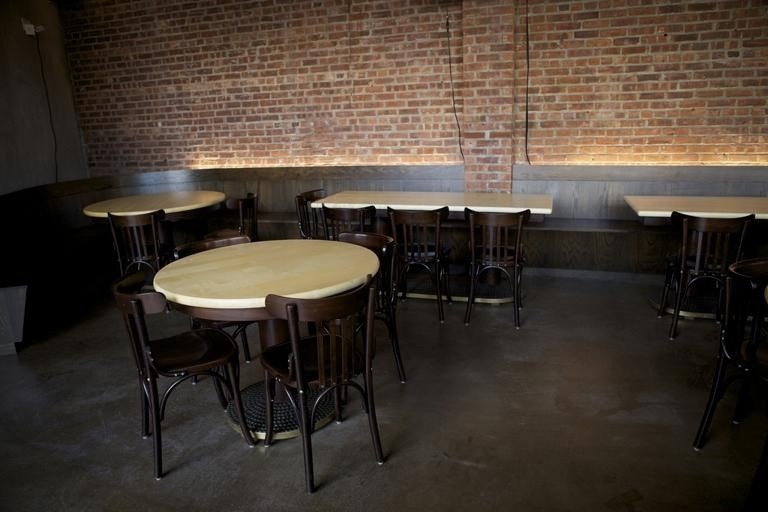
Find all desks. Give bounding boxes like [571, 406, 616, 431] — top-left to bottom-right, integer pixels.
[83, 191, 226, 218]
[623, 196, 768, 219]
[153, 239, 380, 440]
[310, 191, 554, 215]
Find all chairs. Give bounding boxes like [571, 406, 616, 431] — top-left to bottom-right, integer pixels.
[107, 209, 166, 277]
[464, 207, 531, 331]
[259, 273, 385, 496]
[112, 270, 256, 480]
[174, 235, 251, 385]
[655, 211, 755, 341]
[295, 189, 328, 240]
[319, 206, 377, 240]
[211, 197, 258, 242]
[338, 232, 408, 385]
[692, 258, 768, 454]
[387, 206, 453, 324]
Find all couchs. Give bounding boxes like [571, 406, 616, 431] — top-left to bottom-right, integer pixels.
[512, 164, 768, 274]
[0, 165, 464, 356]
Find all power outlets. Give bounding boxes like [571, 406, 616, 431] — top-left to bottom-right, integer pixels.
[21, 16, 44, 36]
